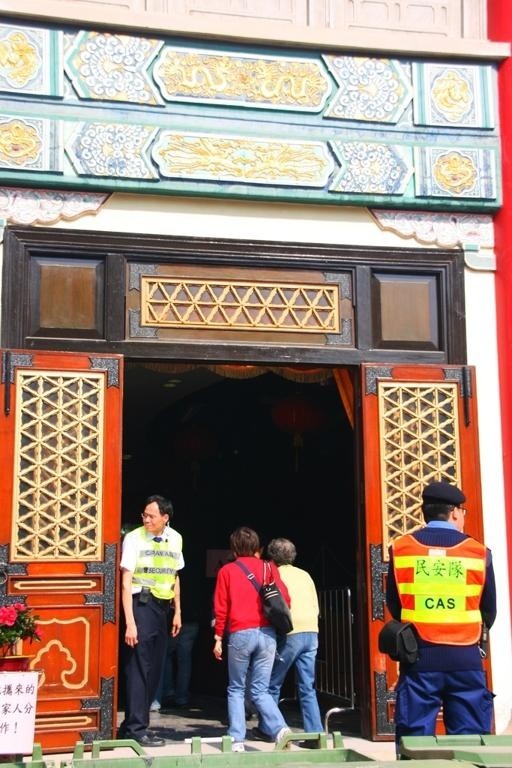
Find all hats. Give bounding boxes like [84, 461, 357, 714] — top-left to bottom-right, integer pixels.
[423, 480, 467, 511]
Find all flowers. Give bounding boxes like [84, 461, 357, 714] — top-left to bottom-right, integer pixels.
[0, 604, 41, 657]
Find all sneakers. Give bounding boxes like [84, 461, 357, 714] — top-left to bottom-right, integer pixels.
[298, 739, 321, 749]
[220, 736, 245, 753]
[275, 728, 292, 751]
[173, 703, 189, 715]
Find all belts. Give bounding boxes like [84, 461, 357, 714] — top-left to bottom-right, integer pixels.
[135, 593, 172, 605]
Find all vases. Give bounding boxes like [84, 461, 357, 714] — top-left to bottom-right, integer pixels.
[1, 654, 35, 672]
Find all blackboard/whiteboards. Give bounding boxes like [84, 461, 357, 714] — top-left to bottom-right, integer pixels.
[0, 671, 39, 755]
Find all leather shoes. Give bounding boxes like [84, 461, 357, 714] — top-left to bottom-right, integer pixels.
[122, 728, 166, 748]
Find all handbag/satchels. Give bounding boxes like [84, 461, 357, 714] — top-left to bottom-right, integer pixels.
[380, 618, 418, 663]
[258, 582, 294, 633]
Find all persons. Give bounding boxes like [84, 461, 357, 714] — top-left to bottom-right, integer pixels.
[211, 526, 294, 751]
[252, 536, 325, 749]
[211, 533, 287, 726]
[385, 481, 497, 759]
[116, 492, 185, 745]
[149, 547, 202, 713]
